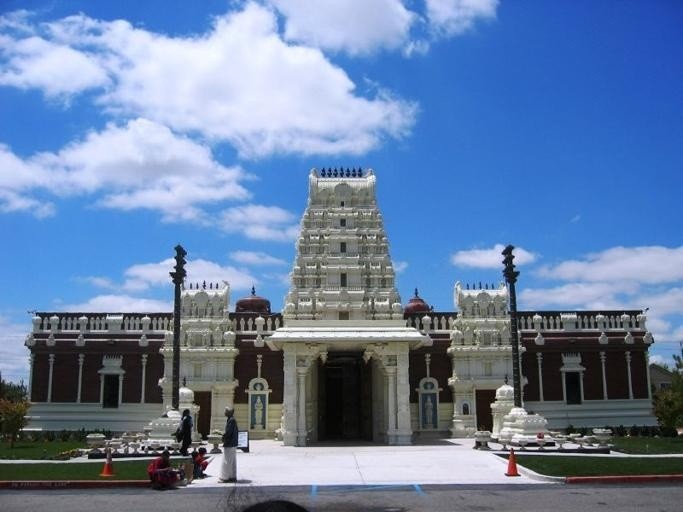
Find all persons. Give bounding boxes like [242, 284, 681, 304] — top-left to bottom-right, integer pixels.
[176, 408, 193, 456]
[146, 450, 179, 490]
[424, 396, 435, 424]
[253, 395, 264, 425]
[217, 406, 238, 483]
[180, 414, 193, 456]
[192, 447, 208, 478]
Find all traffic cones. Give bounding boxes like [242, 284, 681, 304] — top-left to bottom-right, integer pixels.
[98, 448, 114, 477]
[504, 447, 521, 477]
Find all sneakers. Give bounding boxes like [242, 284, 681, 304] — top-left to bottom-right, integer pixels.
[219, 477, 237, 483]
